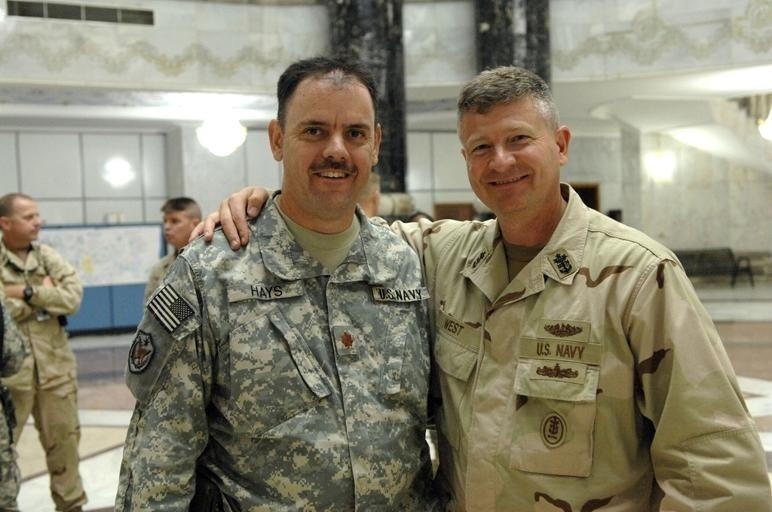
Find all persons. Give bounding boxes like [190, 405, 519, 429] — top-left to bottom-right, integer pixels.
[144, 194, 201, 295]
[1, 192, 92, 512]
[408, 209, 433, 223]
[188, 65, 769, 509]
[470, 211, 497, 222]
[360, 173, 381, 220]
[0, 293, 33, 510]
[114, 52, 435, 510]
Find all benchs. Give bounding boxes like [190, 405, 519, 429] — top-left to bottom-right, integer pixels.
[670, 247, 758, 288]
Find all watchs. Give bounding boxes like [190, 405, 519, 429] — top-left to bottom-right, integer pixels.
[22, 284, 33, 300]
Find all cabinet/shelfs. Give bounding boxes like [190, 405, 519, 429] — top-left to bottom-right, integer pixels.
[62, 280, 146, 339]
